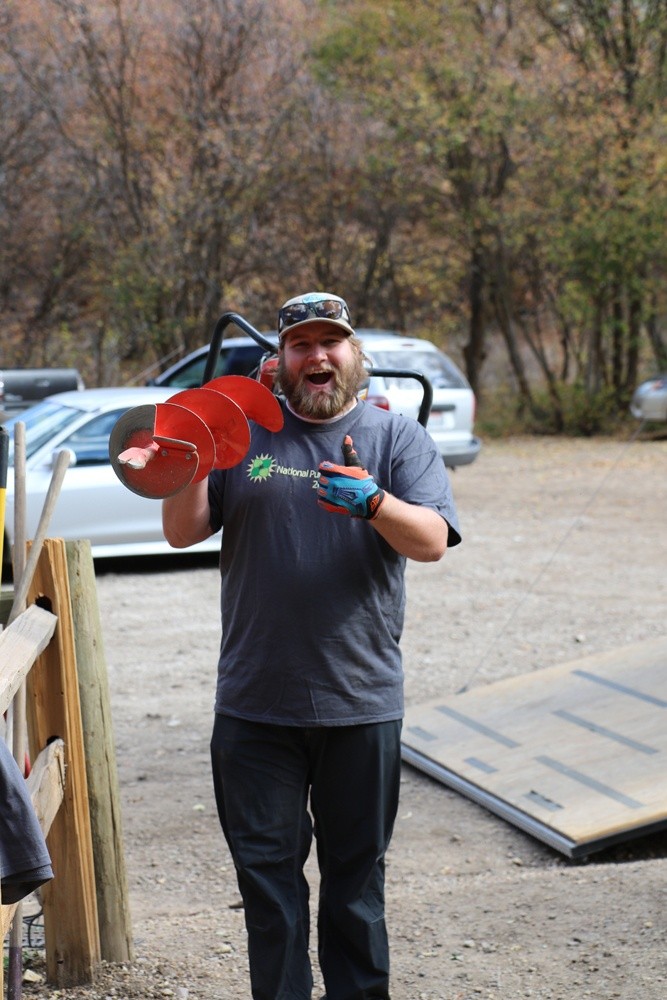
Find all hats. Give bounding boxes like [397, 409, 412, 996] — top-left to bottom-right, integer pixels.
[277, 292, 356, 344]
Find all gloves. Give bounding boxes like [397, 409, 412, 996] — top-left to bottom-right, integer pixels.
[317, 434, 387, 521]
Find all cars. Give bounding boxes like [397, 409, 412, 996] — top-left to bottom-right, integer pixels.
[631, 374, 667, 426]
[0, 386, 227, 575]
[1, 370, 87, 424]
[142, 330, 484, 471]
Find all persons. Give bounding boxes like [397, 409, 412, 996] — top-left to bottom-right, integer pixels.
[160, 291, 464, 1000]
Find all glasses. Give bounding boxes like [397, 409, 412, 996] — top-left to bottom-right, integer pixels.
[278, 299, 351, 333]
